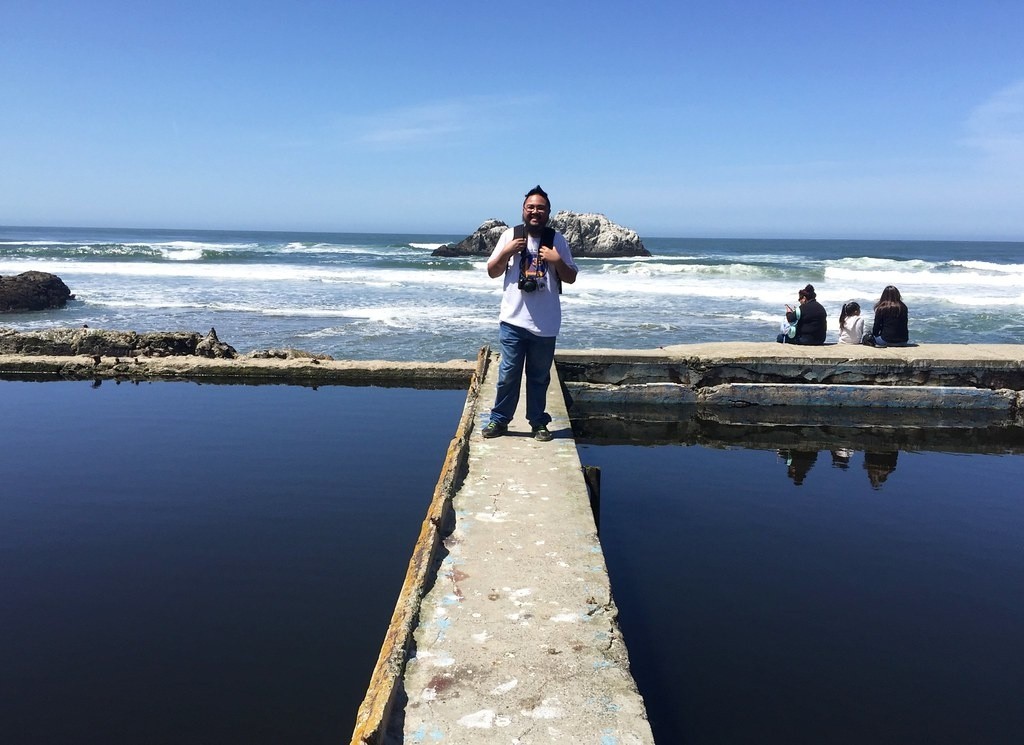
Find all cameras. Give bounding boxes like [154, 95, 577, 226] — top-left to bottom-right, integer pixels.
[518, 276, 538, 293]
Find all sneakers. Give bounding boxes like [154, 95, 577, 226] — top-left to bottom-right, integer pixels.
[530, 424, 552, 441]
[482, 421, 508, 437]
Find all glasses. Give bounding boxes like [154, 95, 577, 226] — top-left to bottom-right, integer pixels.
[798, 297, 804, 301]
[522, 206, 548, 213]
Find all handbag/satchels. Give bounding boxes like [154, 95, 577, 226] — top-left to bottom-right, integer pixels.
[784, 304, 797, 322]
[862, 330, 876, 346]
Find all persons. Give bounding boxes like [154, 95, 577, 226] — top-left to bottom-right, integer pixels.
[776, 284, 827, 346]
[838, 302, 869, 345]
[862, 285, 909, 347]
[481, 185, 579, 440]
